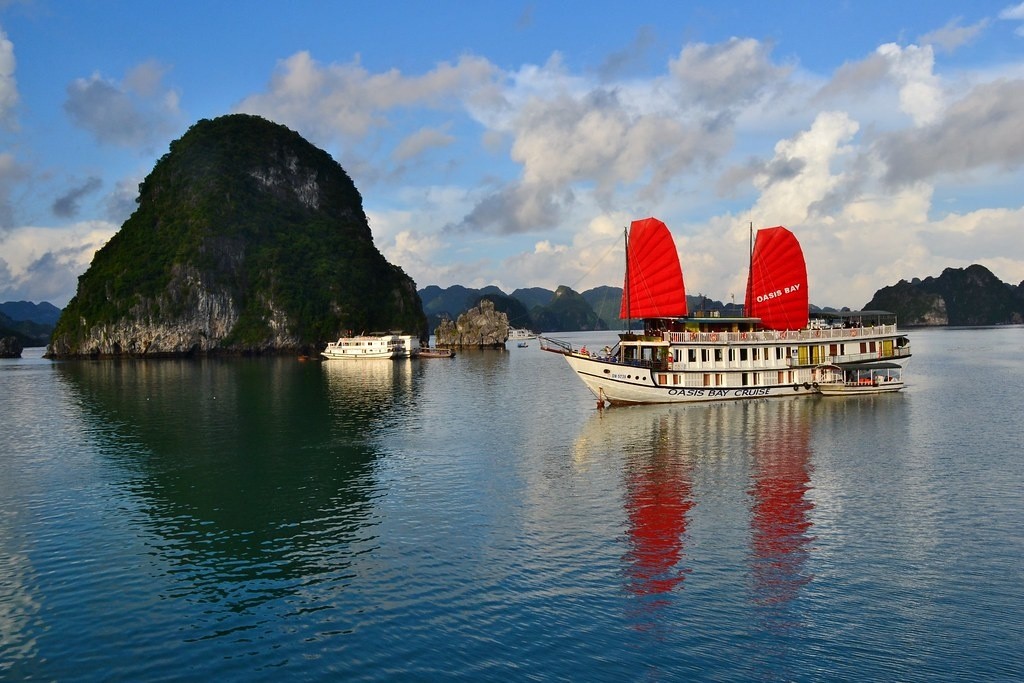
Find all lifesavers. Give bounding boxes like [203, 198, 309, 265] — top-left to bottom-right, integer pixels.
[741, 332, 747, 340]
[780, 332, 787, 339]
[794, 386, 798, 391]
[850, 329, 857, 337]
[813, 382, 818, 387]
[804, 382, 808, 386]
[711, 334, 717, 341]
[815, 331, 821, 337]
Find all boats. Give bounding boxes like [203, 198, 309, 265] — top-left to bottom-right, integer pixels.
[508, 326, 538, 340]
[416, 348, 456, 358]
[517, 342, 529, 348]
[320, 329, 421, 359]
[538, 216, 912, 407]
[807, 363, 904, 396]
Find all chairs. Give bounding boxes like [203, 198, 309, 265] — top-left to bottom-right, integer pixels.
[598, 356, 640, 367]
